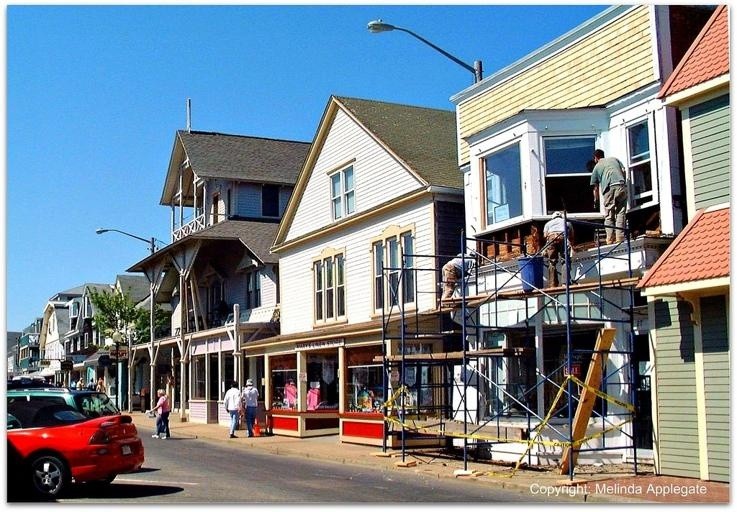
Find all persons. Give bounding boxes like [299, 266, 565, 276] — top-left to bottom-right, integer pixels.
[542, 210, 576, 288]
[589, 148, 628, 245]
[239, 378, 259, 438]
[48, 376, 107, 395]
[145, 388, 172, 439]
[436, 248, 478, 310]
[222, 381, 241, 438]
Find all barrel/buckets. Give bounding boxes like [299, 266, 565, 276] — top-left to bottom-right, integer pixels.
[518, 257, 543, 291]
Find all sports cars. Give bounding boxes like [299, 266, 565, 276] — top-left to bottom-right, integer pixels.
[7, 401, 145, 498]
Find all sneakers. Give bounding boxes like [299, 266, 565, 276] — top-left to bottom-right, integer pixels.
[230, 434, 237, 438]
[151, 435, 169, 440]
[607, 240, 621, 244]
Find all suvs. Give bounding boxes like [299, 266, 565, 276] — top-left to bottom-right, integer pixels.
[7, 375, 121, 418]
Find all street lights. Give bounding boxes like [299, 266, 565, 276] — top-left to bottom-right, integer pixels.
[96, 225, 157, 418]
[112, 328, 122, 412]
[365, 16, 482, 83]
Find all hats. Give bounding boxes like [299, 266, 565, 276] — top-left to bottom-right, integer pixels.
[246, 379, 253, 385]
[551, 212, 562, 218]
